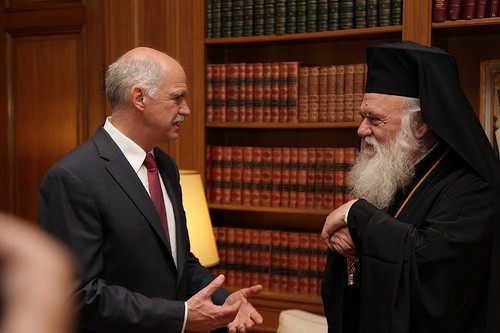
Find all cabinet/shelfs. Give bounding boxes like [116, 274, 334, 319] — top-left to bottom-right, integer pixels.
[190, 0, 500, 333]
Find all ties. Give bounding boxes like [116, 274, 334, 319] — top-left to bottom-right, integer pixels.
[142, 152, 171, 245]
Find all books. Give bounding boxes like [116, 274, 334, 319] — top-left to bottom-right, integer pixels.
[208, 226, 327, 296]
[205, 144, 361, 209]
[479, 59, 500, 157]
[433, 0, 500, 22]
[205, 0, 402, 39]
[205, 61, 369, 122]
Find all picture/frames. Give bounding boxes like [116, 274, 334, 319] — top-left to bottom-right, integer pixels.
[479, 59, 500, 161]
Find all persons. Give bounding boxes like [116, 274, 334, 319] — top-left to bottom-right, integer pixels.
[320, 40, 500, 333]
[38, 47, 262, 333]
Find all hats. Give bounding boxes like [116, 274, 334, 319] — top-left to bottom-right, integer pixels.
[363, 40, 460, 99]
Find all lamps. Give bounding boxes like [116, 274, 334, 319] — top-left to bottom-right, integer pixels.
[180, 169, 219, 270]
